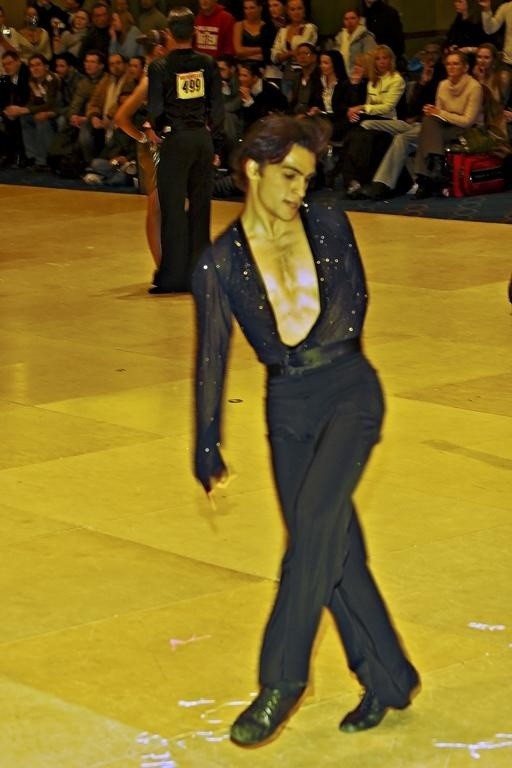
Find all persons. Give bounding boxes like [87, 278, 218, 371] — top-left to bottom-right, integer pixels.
[115, 28, 175, 269]
[146, 6, 224, 297]
[193, 113, 420, 746]
[0, 2, 511, 203]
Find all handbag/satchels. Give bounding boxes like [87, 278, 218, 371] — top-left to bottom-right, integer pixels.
[439, 145, 508, 199]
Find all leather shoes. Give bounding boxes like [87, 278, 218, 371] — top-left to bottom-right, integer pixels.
[338, 673, 422, 732]
[228, 681, 314, 748]
[352, 181, 395, 200]
[148, 285, 190, 294]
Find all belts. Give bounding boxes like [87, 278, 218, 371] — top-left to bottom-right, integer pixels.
[266, 338, 361, 377]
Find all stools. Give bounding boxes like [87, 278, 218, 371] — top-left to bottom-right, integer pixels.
[446, 155, 489, 198]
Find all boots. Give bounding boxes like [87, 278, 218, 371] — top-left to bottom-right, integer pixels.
[413, 153, 447, 179]
[408, 175, 432, 200]
